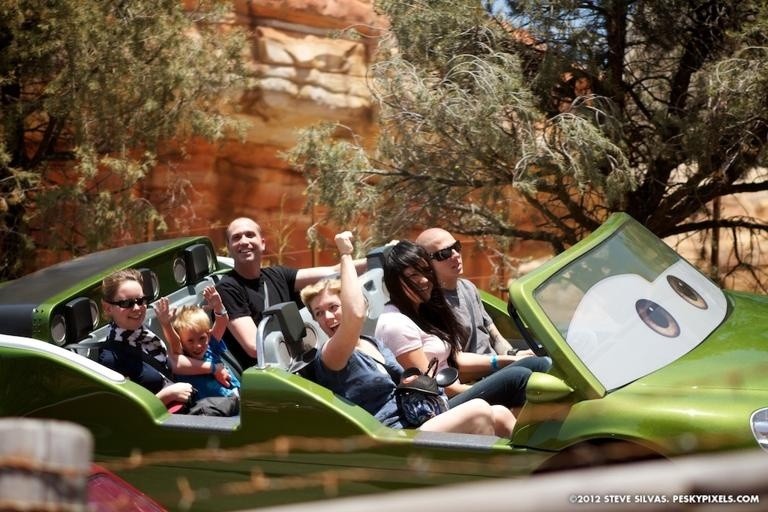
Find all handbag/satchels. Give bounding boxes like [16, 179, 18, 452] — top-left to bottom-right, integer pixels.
[186, 396, 239, 416]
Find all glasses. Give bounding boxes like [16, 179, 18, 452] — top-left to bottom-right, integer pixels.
[103, 298, 143, 308]
[427, 240, 462, 261]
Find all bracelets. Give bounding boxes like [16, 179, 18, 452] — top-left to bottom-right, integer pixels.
[340, 252, 353, 257]
[215, 309, 228, 319]
[490, 353, 498, 370]
[211, 363, 216, 374]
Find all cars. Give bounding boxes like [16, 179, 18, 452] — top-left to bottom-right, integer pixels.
[0, 212, 768, 512]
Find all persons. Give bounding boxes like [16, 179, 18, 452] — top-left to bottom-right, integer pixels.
[212, 217, 368, 369]
[300, 231, 517, 438]
[98, 268, 239, 417]
[415, 227, 536, 380]
[151, 285, 241, 400]
[375, 240, 551, 409]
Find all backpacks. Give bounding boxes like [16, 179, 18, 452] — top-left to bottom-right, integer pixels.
[394, 357, 449, 429]
